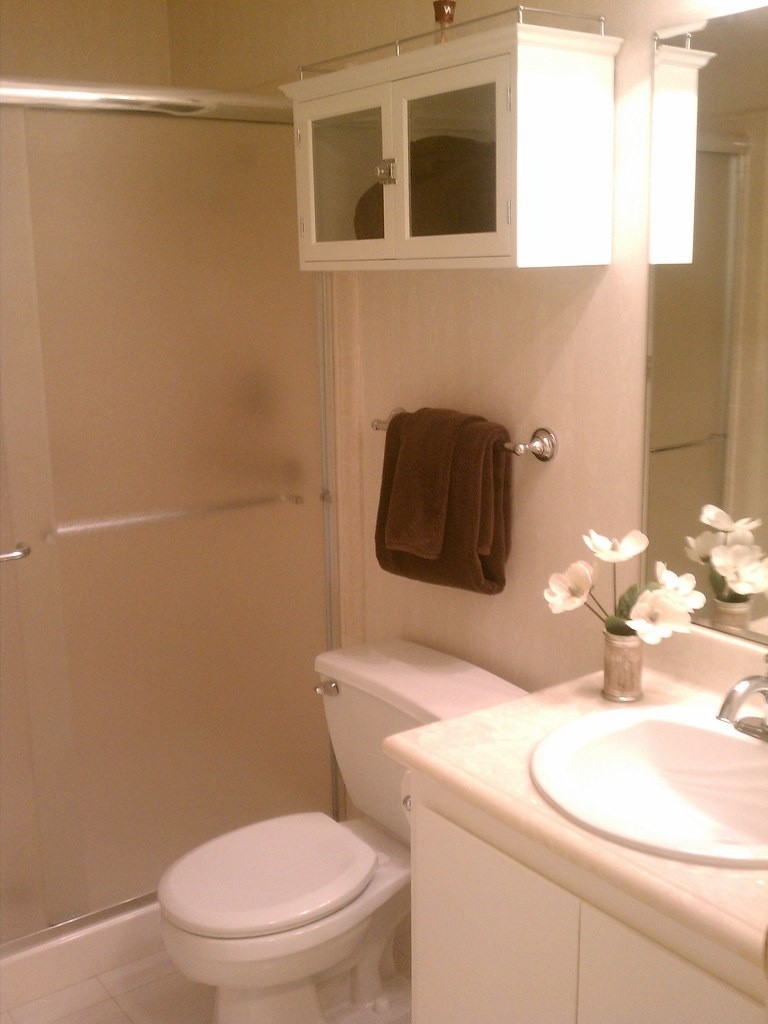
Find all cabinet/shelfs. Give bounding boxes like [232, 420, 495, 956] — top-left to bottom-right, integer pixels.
[405, 769, 766, 1023]
[277, 2, 626, 271]
[645, 31, 717, 266]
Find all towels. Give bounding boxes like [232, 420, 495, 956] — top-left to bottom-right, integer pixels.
[374, 406, 513, 597]
[354, 133, 497, 240]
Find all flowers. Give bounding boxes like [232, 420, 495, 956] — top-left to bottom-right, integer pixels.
[683, 503, 767, 602]
[543, 528, 707, 646]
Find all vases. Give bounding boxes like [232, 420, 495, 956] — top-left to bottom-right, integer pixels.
[599, 629, 645, 703]
[710, 596, 752, 631]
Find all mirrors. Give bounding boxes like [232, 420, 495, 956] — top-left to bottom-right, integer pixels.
[636, 5, 768, 650]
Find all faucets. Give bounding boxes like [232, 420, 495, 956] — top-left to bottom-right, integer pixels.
[718, 652, 768, 725]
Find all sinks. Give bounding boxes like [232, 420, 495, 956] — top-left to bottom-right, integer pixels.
[529, 706, 768, 870]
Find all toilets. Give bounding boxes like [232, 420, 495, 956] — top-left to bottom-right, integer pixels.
[155, 633, 546, 1024]
